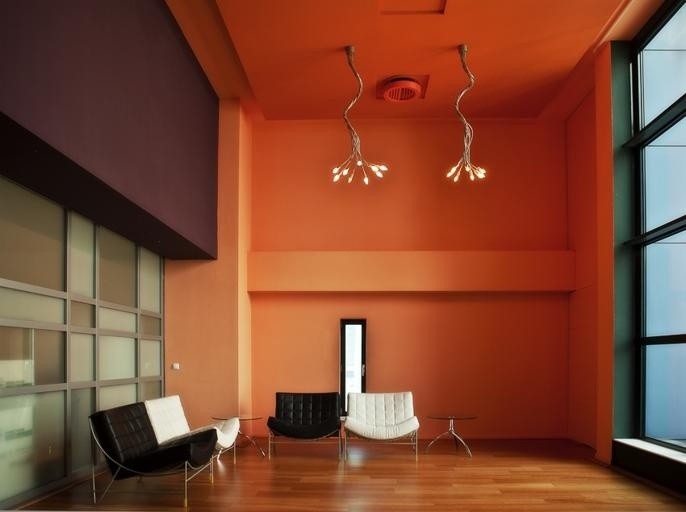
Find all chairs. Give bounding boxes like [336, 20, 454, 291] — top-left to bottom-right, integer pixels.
[266, 391, 420, 462]
[87, 395, 240, 508]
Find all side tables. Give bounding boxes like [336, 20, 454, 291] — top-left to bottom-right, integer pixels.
[211, 415, 266, 459]
[424, 413, 480, 458]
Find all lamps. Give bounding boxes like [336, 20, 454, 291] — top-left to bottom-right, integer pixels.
[332, 44, 388, 185]
[447, 43, 487, 183]
